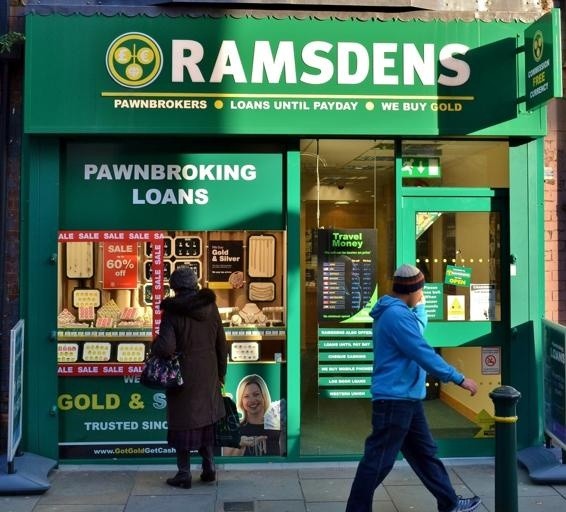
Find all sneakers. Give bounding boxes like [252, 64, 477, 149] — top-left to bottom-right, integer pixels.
[450, 495, 482, 512]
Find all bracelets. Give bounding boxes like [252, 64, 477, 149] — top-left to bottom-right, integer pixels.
[460, 375, 464, 385]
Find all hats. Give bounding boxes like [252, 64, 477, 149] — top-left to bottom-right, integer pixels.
[170, 266, 198, 289]
[392, 263, 425, 294]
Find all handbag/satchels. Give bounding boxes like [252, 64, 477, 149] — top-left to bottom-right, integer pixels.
[140, 346, 185, 392]
[216, 382, 242, 450]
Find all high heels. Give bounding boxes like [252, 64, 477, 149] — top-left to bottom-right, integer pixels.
[200, 471, 216, 483]
[166, 472, 192, 489]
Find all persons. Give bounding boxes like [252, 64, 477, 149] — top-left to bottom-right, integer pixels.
[223, 374, 285, 456]
[150, 263, 228, 488]
[345, 263, 482, 512]
